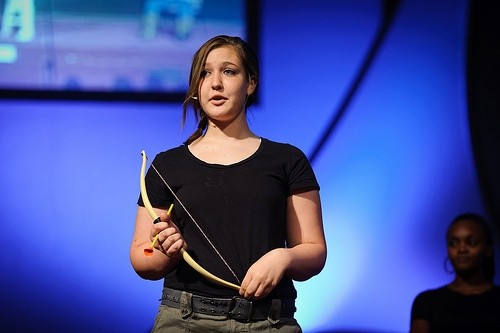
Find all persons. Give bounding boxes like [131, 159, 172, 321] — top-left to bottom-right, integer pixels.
[129, 34, 327, 333]
[409, 212, 500, 333]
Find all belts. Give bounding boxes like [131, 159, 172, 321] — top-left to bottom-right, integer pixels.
[160, 287, 297, 318]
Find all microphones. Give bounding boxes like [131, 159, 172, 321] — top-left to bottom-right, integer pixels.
[192, 97, 198, 99]
